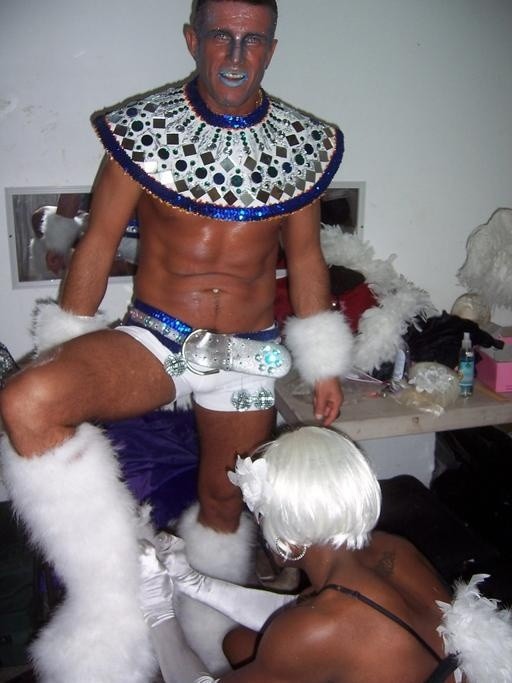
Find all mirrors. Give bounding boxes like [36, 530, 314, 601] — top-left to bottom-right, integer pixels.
[4, 179, 365, 291]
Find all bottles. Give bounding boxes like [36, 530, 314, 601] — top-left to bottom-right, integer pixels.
[458, 332, 476, 399]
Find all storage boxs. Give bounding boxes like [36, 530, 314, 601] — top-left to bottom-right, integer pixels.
[472, 335, 512, 393]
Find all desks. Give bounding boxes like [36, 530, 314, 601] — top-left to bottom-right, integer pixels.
[269, 352, 512, 438]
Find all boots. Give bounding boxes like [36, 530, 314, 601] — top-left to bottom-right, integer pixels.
[176, 504, 257, 675]
[2, 428, 170, 683]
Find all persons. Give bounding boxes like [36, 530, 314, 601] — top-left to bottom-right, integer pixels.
[122, 419, 509, 681]
[46, 194, 82, 272]
[1, 0, 351, 682]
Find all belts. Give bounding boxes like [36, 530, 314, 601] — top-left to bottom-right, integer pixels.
[124, 306, 293, 382]
